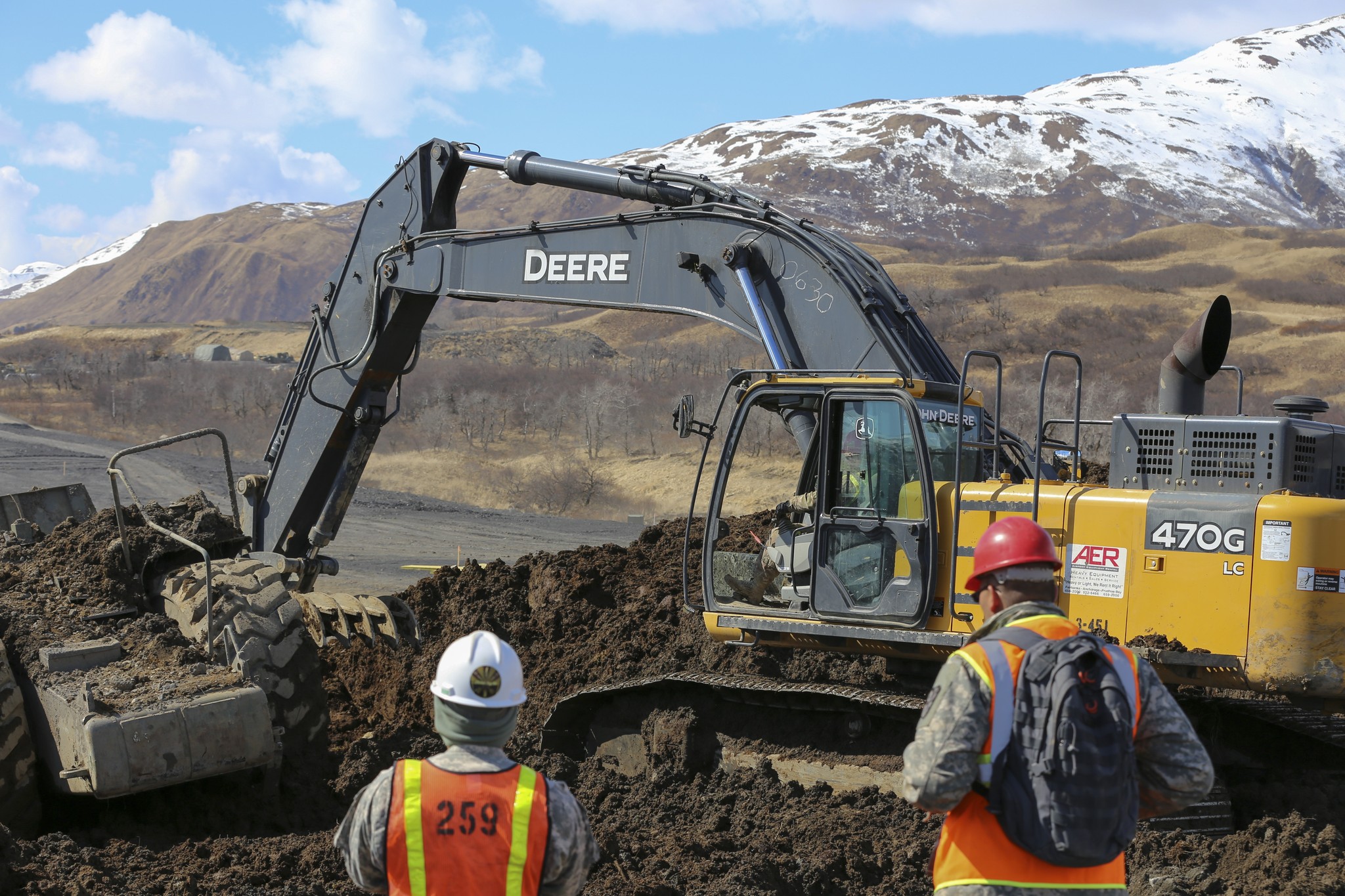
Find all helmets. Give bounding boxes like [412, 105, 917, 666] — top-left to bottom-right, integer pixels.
[964, 516, 1063, 591]
[430, 631, 529, 707]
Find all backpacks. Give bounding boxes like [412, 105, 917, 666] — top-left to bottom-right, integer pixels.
[970, 625, 1139, 866]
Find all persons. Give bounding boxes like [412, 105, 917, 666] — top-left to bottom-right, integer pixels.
[333, 630, 600, 896]
[904, 517, 1214, 896]
[775, 430, 885, 517]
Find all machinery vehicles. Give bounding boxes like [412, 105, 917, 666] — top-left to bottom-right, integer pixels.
[232, 138, 1345, 845]
